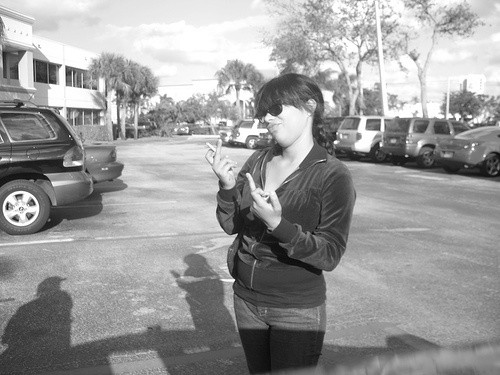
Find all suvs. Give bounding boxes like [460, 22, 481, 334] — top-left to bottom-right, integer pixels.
[315, 115, 343, 153]
[0, 85, 94, 235]
[334, 116, 392, 162]
[382, 118, 470, 169]
[230, 118, 276, 149]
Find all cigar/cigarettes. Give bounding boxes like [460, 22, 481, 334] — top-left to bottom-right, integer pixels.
[206, 142, 217, 154]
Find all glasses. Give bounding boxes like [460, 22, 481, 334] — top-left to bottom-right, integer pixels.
[258, 102, 306, 124]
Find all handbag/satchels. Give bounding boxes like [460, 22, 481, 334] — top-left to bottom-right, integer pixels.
[226, 229, 245, 278]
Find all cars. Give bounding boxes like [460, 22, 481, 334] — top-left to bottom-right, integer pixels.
[430, 125, 500, 178]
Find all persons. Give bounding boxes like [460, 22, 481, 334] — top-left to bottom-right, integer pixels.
[204, 73, 356, 375]
